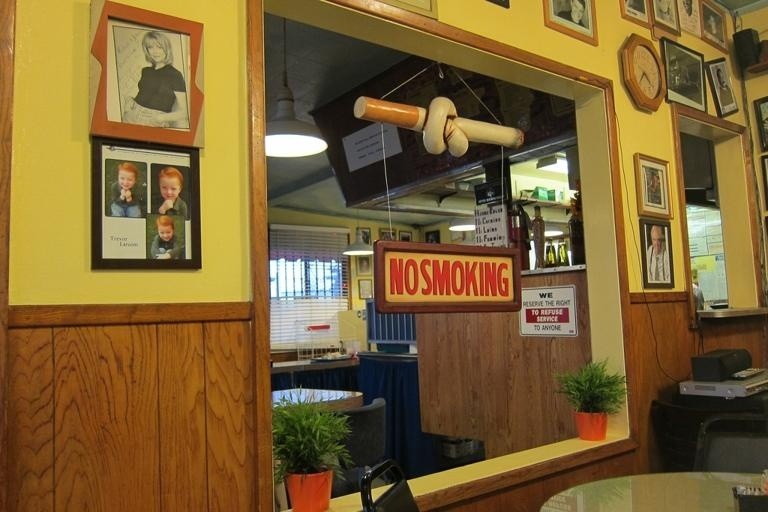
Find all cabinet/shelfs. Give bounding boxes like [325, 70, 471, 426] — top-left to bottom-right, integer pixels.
[269, 355, 360, 390]
[355, 350, 485, 480]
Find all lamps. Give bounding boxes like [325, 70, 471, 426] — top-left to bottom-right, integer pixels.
[265, 17, 328, 159]
[536, 152, 569, 176]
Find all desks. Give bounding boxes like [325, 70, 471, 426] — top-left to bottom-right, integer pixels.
[414, 264, 592, 460]
[539, 469, 768, 512]
[650, 390, 768, 472]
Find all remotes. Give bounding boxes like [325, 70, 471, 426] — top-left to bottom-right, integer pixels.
[730, 367, 765, 379]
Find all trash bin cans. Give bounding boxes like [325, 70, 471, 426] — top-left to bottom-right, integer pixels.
[441, 437, 485, 469]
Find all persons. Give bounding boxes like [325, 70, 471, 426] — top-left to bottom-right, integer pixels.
[716, 68, 733, 105]
[681, 0, 697, 33]
[708, 14, 719, 38]
[427, 234, 437, 243]
[155, 167, 189, 216]
[151, 217, 186, 259]
[558, 0, 586, 27]
[110, 162, 141, 219]
[647, 171, 661, 205]
[124, 31, 188, 128]
[647, 225, 670, 281]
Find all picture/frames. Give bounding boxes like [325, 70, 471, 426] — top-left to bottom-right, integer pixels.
[91, 137, 202, 274]
[89, 0, 204, 149]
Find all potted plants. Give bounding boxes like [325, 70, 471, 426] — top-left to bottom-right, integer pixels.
[551, 355, 632, 441]
[272, 383, 357, 512]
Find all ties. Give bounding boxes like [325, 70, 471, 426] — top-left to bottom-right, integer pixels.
[653, 255, 662, 281]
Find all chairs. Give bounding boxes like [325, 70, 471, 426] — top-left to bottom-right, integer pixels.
[359, 457, 422, 512]
[693, 412, 768, 473]
[332, 398, 388, 468]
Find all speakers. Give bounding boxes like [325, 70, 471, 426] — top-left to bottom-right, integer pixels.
[733, 28, 761, 69]
[691, 349, 752, 382]
